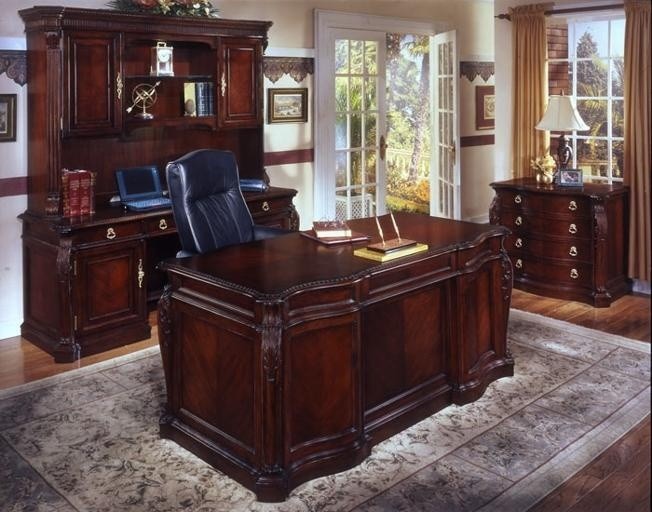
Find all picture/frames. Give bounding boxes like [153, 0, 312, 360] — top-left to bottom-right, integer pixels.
[266, 87, 308, 125]
[557, 168, 584, 188]
[474, 85, 495, 130]
[0, 93, 18, 143]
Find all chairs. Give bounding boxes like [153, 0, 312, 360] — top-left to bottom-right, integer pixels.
[164, 147, 300, 259]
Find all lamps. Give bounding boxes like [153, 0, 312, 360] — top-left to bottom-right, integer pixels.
[531, 88, 591, 185]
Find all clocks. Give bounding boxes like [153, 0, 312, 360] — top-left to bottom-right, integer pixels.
[149, 42, 174, 76]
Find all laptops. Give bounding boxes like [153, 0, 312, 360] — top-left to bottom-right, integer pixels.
[114, 164, 172, 212]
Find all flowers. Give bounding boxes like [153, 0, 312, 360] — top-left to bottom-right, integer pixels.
[108, 0, 222, 19]
[530, 152, 557, 171]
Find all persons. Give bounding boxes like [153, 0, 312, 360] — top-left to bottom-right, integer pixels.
[561, 171, 578, 182]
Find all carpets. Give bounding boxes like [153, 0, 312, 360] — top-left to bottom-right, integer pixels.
[3, 306, 650, 512]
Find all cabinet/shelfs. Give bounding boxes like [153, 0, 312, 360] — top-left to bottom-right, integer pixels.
[481, 175, 635, 308]
[16, 5, 300, 211]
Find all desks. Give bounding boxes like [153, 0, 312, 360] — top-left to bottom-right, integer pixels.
[15, 185, 301, 364]
[149, 208, 518, 502]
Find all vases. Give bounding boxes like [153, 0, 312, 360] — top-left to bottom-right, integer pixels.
[535, 166, 553, 184]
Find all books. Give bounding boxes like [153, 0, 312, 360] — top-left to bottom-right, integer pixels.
[301, 219, 370, 244]
[183, 81, 216, 117]
[61, 168, 96, 218]
